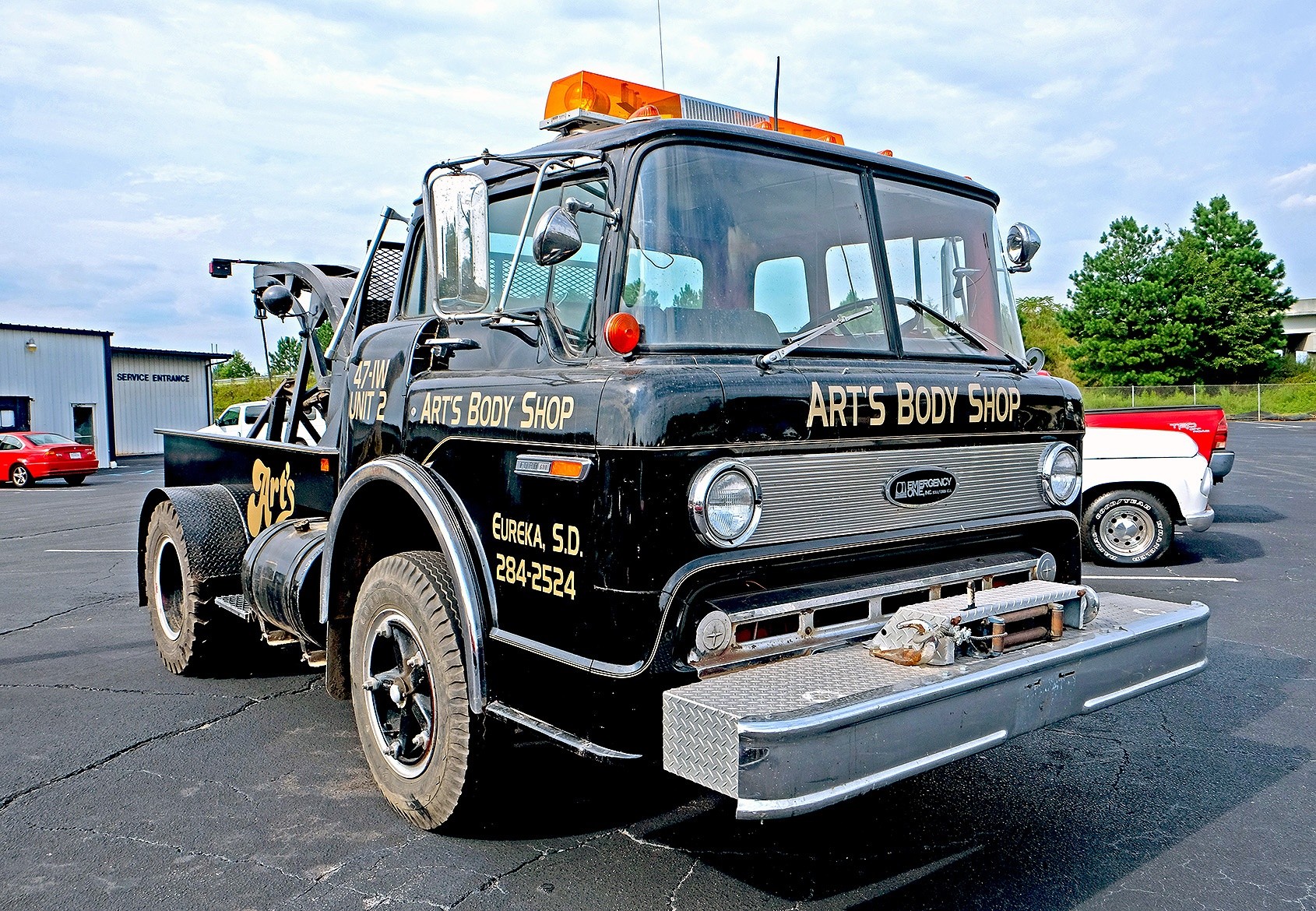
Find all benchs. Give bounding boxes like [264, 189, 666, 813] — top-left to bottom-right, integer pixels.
[505, 299, 787, 345]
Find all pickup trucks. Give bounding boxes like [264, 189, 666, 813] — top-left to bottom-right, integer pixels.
[133, 69, 1213, 835]
[1038, 370, 1235, 483]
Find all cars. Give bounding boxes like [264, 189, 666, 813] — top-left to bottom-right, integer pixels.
[1070, 426, 1216, 568]
[0, 432, 99, 488]
[194, 398, 326, 448]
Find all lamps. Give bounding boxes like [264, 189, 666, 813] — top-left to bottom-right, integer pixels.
[24, 338, 37, 353]
